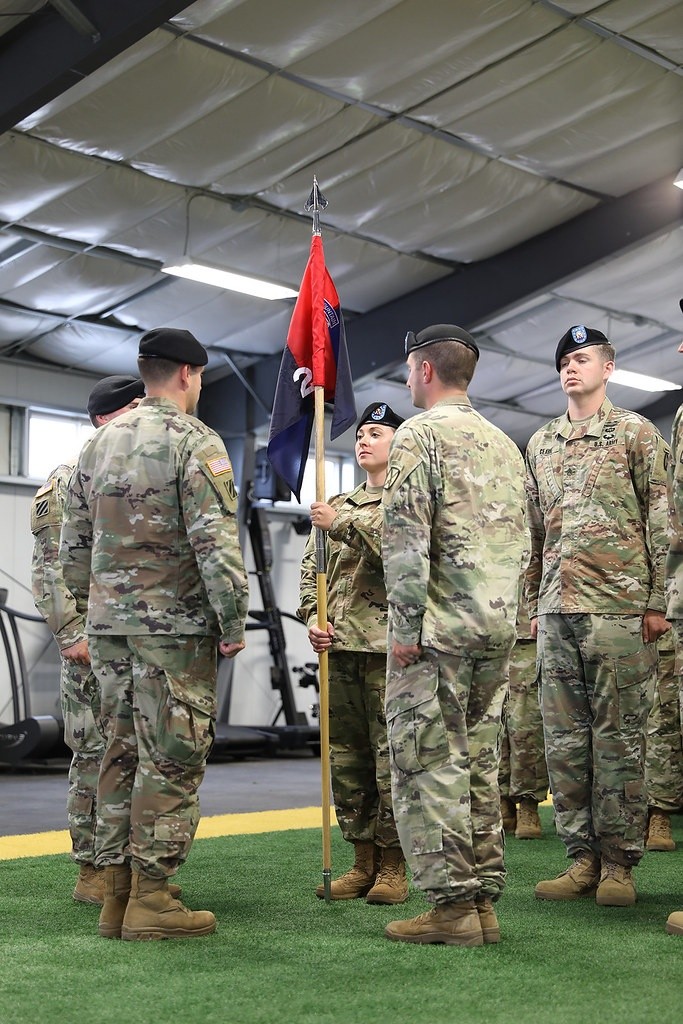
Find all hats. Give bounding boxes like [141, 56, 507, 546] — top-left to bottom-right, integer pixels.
[138, 327, 209, 367]
[87, 375, 145, 415]
[556, 325, 611, 373]
[356, 402, 405, 437]
[405, 324, 480, 361]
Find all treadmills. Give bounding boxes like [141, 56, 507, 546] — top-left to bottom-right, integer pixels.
[1, 477, 324, 767]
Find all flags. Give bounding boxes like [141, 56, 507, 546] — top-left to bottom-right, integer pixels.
[266, 231, 357, 504]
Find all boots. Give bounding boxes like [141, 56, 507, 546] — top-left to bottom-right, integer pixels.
[535, 852, 638, 906]
[72, 863, 184, 903]
[316, 843, 411, 904]
[644, 813, 676, 851]
[502, 798, 542, 839]
[384, 896, 501, 947]
[97, 866, 218, 940]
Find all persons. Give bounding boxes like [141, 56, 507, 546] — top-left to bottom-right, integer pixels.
[297, 403, 410, 901]
[381, 324, 532, 947]
[498, 575, 550, 839]
[526, 325, 672, 910]
[58, 327, 247, 938]
[644, 300, 683, 935]
[32, 374, 183, 906]
